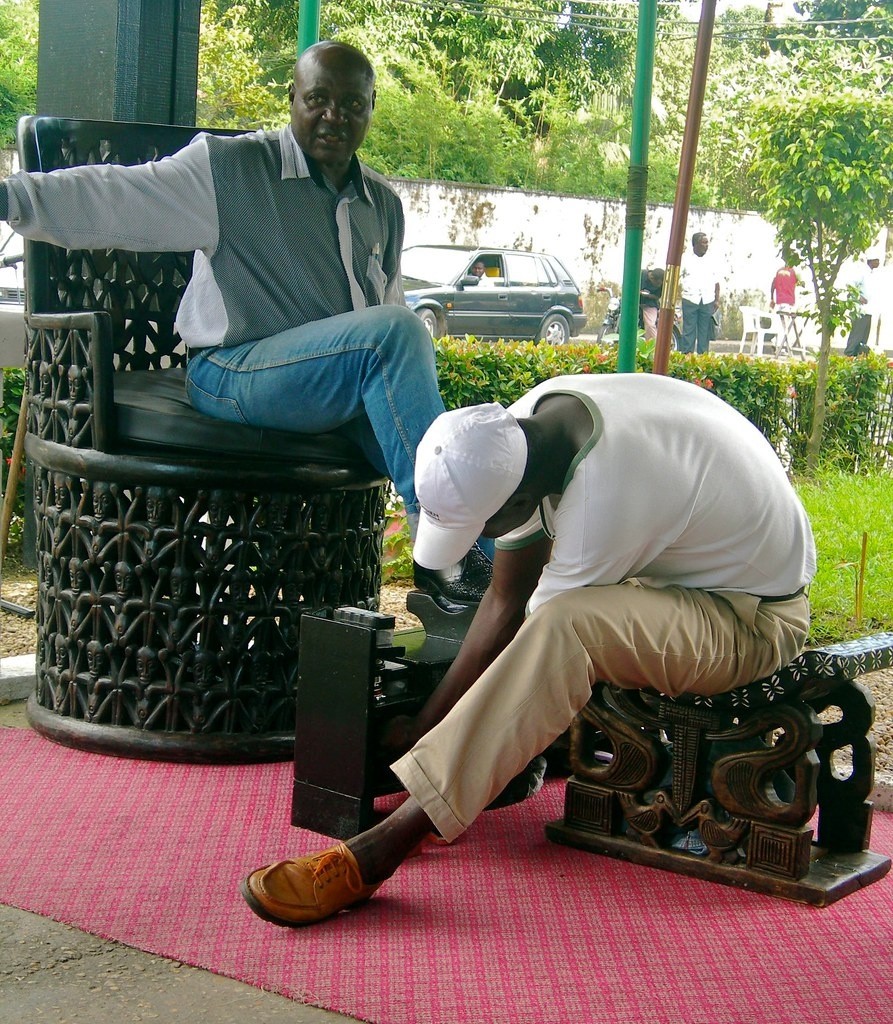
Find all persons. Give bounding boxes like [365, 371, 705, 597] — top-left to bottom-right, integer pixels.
[843, 254, 881, 357]
[240, 374, 818, 929]
[0, 41, 498, 603]
[675, 232, 720, 352]
[463, 260, 491, 285]
[769, 248, 805, 357]
[638, 268, 667, 338]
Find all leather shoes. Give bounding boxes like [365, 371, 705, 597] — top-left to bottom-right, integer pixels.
[239, 840, 385, 929]
[413, 541, 494, 607]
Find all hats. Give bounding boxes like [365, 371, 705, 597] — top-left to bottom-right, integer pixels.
[412, 402, 527, 571]
[864, 246, 886, 260]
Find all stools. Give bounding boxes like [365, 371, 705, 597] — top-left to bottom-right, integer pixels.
[547, 631, 893, 908]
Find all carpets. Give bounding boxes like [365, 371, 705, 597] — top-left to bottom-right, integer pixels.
[2, 730, 893, 1024]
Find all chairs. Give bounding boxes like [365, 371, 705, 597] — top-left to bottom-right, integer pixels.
[10, 115, 391, 765]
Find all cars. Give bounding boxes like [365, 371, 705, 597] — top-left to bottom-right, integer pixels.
[400, 245, 588, 347]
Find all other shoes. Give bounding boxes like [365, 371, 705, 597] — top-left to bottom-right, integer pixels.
[774, 350, 789, 357]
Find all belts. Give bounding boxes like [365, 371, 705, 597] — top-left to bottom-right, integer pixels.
[189, 346, 220, 361]
[746, 586, 806, 604]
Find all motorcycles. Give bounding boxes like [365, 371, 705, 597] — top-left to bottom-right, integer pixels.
[597, 288, 682, 351]
